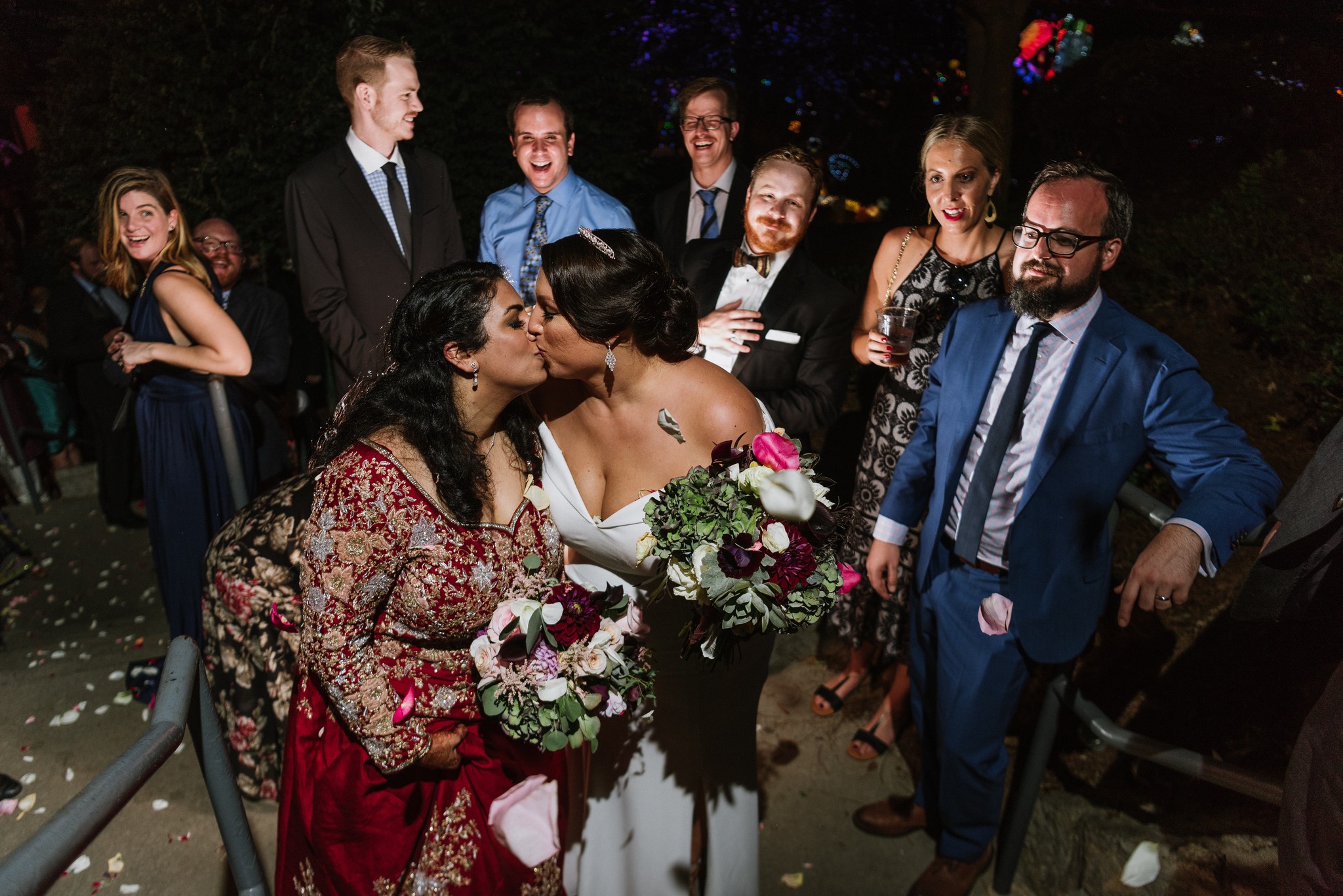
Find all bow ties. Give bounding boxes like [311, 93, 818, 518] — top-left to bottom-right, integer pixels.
[732, 246, 771, 278]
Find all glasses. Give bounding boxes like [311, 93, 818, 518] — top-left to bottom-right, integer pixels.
[197, 236, 243, 255]
[680, 115, 731, 131]
[1011, 225, 1108, 256]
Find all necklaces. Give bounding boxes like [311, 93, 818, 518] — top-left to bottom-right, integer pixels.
[472, 428, 495, 479]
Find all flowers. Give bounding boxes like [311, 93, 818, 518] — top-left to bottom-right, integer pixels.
[469, 556, 657, 752]
[636, 427, 862, 673]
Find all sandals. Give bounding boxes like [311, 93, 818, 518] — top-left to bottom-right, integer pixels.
[809, 668, 868, 714]
[850, 704, 904, 760]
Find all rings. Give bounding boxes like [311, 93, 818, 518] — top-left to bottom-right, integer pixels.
[1155, 595, 1169, 601]
[118, 361, 123, 366]
[732, 330, 736, 338]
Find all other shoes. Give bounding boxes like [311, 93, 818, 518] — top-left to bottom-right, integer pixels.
[108, 513, 148, 531]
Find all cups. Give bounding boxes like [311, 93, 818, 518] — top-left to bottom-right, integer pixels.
[874, 306, 920, 366]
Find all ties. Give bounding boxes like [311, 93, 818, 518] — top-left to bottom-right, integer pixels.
[380, 162, 413, 272]
[698, 189, 718, 238]
[519, 195, 554, 307]
[92, 288, 109, 311]
[954, 323, 1055, 567]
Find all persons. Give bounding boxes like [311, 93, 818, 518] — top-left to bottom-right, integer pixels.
[812, 115, 1021, 760]
[0, 286, 94, 471]
[203, 466, 324, 802]
[54, 239, 147, 529]
[475, 88, 636, 316]
[271, 262, 565, 895]
[286, 34, 464, 412]
[528, 225, 774, 896]
[192, 218, 288, 497]
[654, 76, 751, 268]
[674, 146, 852, 439]
[852, 154, 1280, 896]
[100, 168, 250, 653]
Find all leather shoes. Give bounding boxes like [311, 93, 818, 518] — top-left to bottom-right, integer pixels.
[853, 794, 925, 835]
[0, 774, 23, 800]
[909, 842, 993, 896]
